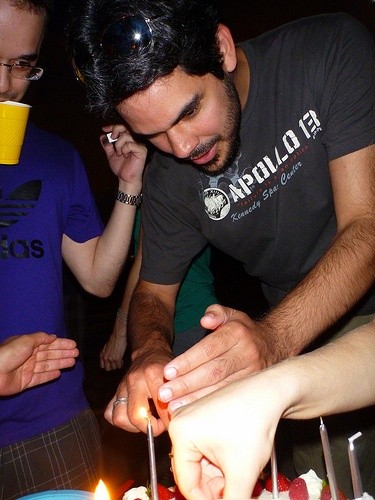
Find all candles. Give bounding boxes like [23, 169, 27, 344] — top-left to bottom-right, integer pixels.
[348, 430, 365, 500]
[144, 408, 159, 500]
[318, 416, 341, 500]
[269, 441, 279, 500]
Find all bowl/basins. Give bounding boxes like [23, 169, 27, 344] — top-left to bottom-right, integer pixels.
[15, 489, 93, 500]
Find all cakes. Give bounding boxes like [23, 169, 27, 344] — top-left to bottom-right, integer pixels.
[116, 469, 375, 500]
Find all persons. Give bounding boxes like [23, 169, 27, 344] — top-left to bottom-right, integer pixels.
[75, 0, 375, 500]
[0, 331, 79, 398]
[168, 315, 375, 500]
[100, 205, 216, 373]
[1, 1, 146, 498]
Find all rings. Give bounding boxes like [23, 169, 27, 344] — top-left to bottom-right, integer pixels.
[113, 397, 127, 407]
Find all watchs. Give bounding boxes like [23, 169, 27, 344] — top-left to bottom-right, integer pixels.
[115, 190, 144, 207]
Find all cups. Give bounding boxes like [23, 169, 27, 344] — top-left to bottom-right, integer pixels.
[0, 100, 32, 165]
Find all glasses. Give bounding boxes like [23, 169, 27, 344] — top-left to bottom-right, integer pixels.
[0, 62, 46, 81]
